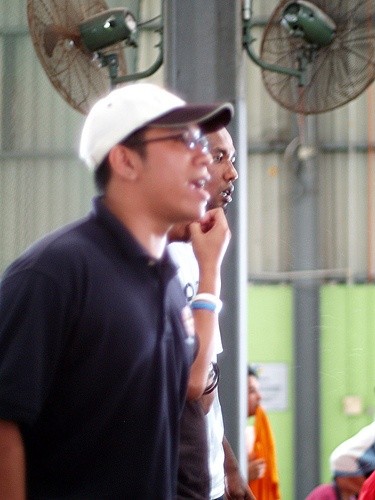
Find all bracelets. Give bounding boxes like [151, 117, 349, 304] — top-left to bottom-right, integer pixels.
[189, 301, 216, 310]
[192, 293, 222, 313]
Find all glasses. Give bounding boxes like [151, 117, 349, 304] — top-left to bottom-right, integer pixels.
[129, 130, 208, 152]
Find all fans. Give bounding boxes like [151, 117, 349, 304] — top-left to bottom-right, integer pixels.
[242, 0, 375, 115]
[27, 0, 163, 116]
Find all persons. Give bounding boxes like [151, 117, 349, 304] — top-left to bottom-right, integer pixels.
[248, 366, 281, 500]
[0, 84, 256, 500]
[305, 422, 375, 500]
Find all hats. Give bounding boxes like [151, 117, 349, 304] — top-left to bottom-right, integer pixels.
[79, 83, 234, 172]
